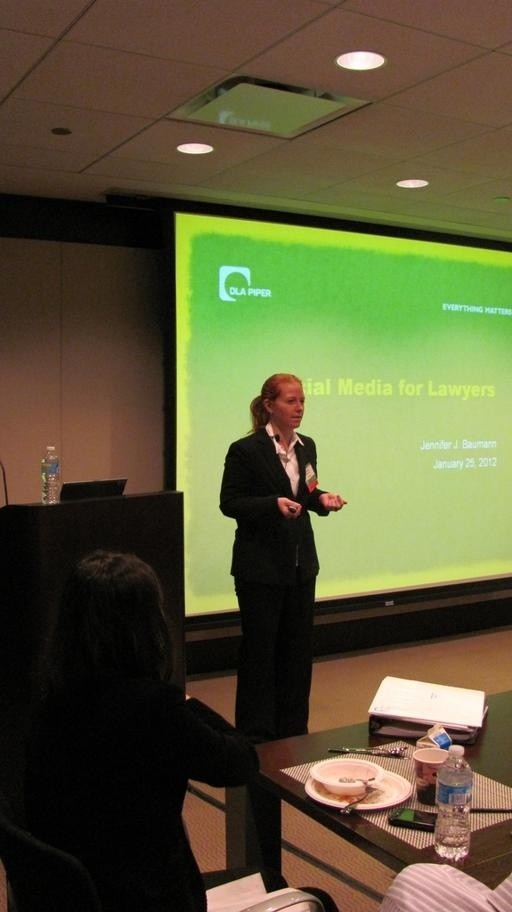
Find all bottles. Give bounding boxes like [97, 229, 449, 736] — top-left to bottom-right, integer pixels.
[39, 445, 61, 506]
[432, 744, 474, 860]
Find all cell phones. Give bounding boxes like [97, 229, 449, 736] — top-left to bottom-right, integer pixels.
[388, 807, 438, 833]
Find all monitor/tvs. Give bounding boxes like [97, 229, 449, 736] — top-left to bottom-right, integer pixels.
[59, 478, 128, 501]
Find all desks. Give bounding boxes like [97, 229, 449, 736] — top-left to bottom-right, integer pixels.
[225, 687, 510, 912]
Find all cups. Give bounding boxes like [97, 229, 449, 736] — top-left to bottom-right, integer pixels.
[412, 747, 448, 805]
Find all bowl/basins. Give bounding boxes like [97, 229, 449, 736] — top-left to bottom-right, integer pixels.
[308, 756, 384, 798]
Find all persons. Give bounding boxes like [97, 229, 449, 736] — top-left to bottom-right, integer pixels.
[24, 549, 260, 910]
[221, 371, 349, 740]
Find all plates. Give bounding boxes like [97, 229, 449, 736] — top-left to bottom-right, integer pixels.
[303, 769, 414, 811]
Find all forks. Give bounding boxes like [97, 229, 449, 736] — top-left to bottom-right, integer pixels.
[340, 785, 380, 820]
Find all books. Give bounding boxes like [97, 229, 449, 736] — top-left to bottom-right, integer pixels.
[368, 672, 487, 730]
[364, 670, 490, 746]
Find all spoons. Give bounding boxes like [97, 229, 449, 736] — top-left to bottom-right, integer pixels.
[322, 746, 413, 754]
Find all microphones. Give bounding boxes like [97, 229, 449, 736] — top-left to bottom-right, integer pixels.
[271, 434, 280, 442]
